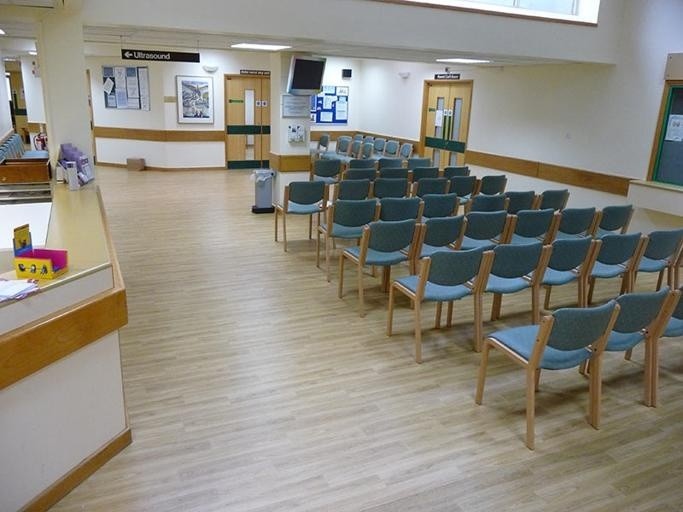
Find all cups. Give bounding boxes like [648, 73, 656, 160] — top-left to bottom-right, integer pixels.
[68, 168, 79, 191]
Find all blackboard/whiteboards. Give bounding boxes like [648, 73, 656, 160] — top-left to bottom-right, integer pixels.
[652, 85, 683, 187]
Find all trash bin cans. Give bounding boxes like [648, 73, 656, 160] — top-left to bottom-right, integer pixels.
[255, 170, 274, 208]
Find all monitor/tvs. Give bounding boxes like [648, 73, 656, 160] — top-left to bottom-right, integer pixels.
[286, 56, 326, 96]
[342, 69, 350, 78]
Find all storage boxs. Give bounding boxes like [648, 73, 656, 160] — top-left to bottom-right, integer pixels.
[126, 158, 146, 172]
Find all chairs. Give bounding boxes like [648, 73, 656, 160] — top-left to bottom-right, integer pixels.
[587, 284, 675, 427]
[492, 208, 557, 248]
[620, 229, 682, 294]
[378, 166, 410, 182]
[421, 192, 460, 220]
[579, 205, 635, 235]
[496, 233, 596, 324]
[452, 209, 511, 251]
[378, 157, 405, 168]
[373, 137, 385, 157]
[338, 217, 421, 317]
[364, 136, 375, 145]
[383, 140, 401, 157]
[310, 134, 330, 156]
[624, 286, 683, 408]
[386, 245, 489, 363]
[440, 166, 471, 179]
[451, 175, 477, 197]
[360, 143, 375, 159]
[348, 158, 377, 168]
[674, 248, 682, 287]
[399, 143, 415, 157]
[386, 214, 466, 310]
[476, 175, 507, 195]
[309, 158, 346, 181]
[270, 180, 328, 253]
[372, 178, 411, 199]
[540, 206, 600, 241]
[325, 136, 351, 160]
[352, 133, 364, 141]
[341, 169, 377, 181]
[464, 195, 511, 214]
[405, 158, 433, 167]
[347, 140, 362, 158]
[505, 190, 537, 214]
[474, 300, 621, 452]
[549, 231, 645, 309]
[412, 177, 450, 197]
[0, 134, 49, 158]
[378, 196, 423, 222]
[537, 188, 571, 210]
[410, 167, 441, 183]
[316, 179, 372, 237]
[315, 198, 378, 284]
[446, 240, 549, 354]
